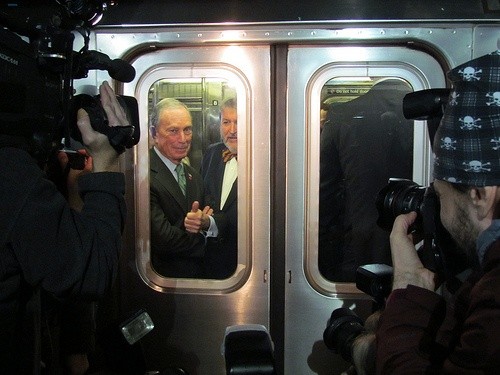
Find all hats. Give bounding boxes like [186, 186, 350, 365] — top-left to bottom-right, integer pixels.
[432, 50, 500, 187]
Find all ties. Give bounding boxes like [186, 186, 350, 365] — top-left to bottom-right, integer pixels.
[174, 164, 187, 197]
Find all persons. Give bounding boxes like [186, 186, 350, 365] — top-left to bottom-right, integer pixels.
[318, 79, 413, 283]
[0, 28, 128, 375]
[374, 53, 500, 375]
[128, 98, 238, 279]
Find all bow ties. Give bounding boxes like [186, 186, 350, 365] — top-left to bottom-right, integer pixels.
[222, 147, 237, 163]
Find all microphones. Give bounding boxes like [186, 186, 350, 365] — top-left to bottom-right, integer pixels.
[107, 58, 136, 83]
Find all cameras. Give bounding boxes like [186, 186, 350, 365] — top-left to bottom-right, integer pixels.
[374, 90, 454, 252]
[324, 261, 395, 366]
